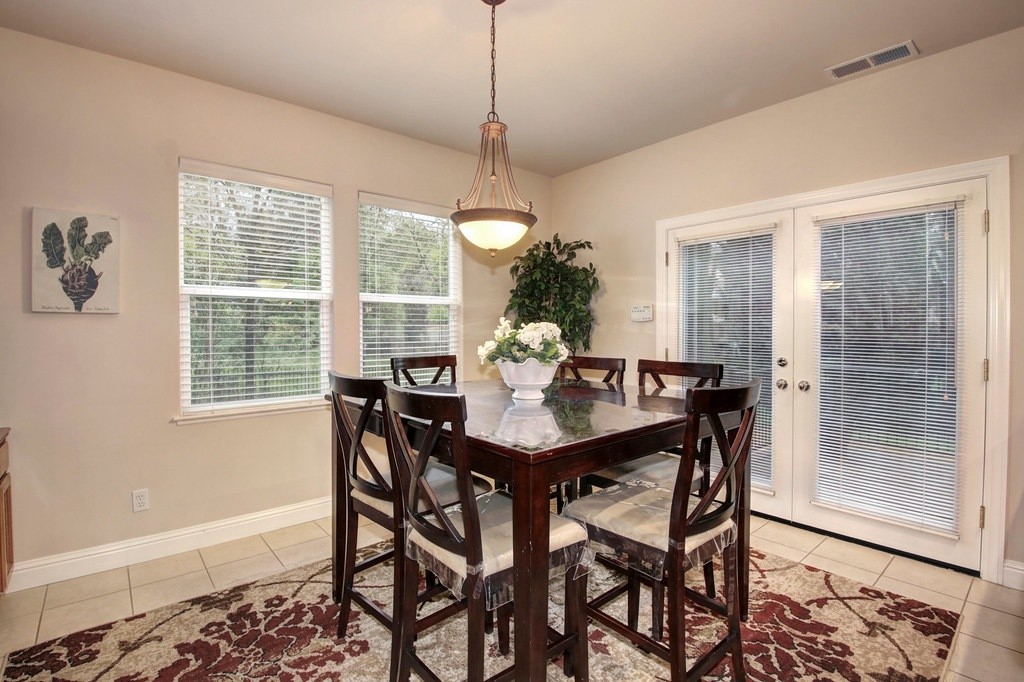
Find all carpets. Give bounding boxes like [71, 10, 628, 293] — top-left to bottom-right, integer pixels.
[0, 482, 960, 682]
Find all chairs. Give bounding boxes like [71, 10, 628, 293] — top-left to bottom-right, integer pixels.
[390, 357, 458, 392]
[385, 384, 591, 682]
[551, 356, 626, 515]
[578, 358, 724, 513]
[561, 379, 763, 682]
[328, 367, 492, 639]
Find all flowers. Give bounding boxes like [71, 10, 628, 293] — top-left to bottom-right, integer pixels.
[478, 317, 568, 367]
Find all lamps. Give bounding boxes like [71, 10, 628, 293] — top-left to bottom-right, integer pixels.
[450, 0, 536, 255]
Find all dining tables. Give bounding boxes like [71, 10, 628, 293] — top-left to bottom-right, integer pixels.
[323, 377, 745, 682]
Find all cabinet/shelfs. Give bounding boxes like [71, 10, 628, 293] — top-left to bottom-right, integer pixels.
[0, 427, 14, 596]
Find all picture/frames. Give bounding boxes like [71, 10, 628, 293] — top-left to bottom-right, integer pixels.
[31, 206, 120, 314]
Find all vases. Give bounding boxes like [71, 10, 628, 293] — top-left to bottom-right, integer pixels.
[495, 407, 564, 448]
[494, 359, 560, 400]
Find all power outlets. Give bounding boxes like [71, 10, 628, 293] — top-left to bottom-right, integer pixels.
[131, 488, 149, 512]
